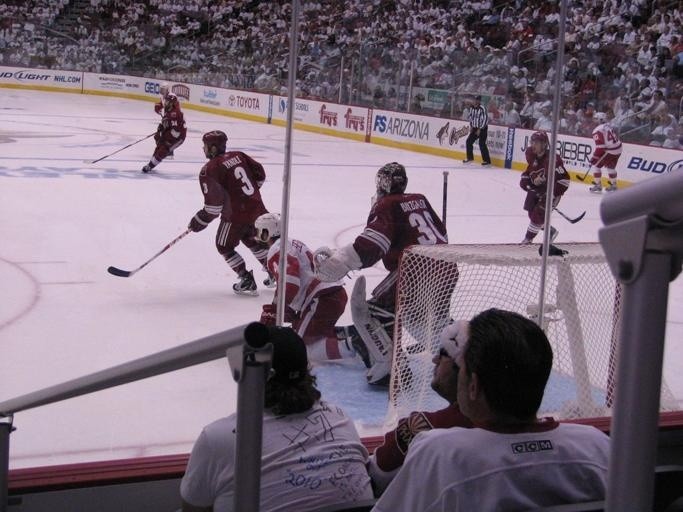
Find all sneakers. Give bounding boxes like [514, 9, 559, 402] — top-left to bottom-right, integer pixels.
[143, 166, 152, 172]
[343, 325, 372, 368]
[482, 160, 490, 164]
[463, 158, 473, 162]
[550, 226, 556, 239]
[520, 238, 533, 246]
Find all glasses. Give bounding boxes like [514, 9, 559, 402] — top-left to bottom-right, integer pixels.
[439, 348, 449, 357]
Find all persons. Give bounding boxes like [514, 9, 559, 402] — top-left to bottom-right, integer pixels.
[373, 308, 611, 512]
[314, 162, 460, 389]
[183, 327, 377, 511]
[1, 1, 683, 190]
[520, 129, 570, 243]
[187, 130, 277, 291]
[253, 213, 372, 370]
[365, 319, 477, 496]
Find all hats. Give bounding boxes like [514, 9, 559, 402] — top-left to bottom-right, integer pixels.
[245, 319, 307, 379]
[441, 320, 470, 369]
[473, 95, 482, 100]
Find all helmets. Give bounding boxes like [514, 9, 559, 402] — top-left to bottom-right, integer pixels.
[203, 130, 227, 149]
[531, 132, 548, 143]
[594, 112, 606, 119]
[376, 162, 407, 202]
[164, 93, 178, 112]
[160, 83, 170, 95]
[255, 213, 281, 243]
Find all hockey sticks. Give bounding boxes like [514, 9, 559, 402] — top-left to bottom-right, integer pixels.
[576, 165, 591, 181]
[406, 171, 449, 354]
[83, 132, 159, 165]
[529, 183, 586, 223]
[107, 228, 193, 277]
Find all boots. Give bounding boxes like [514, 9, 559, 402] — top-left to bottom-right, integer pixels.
[233, 270, 257, 290]
[606, 181, 617, 191]
[590, 181, 603, 191]
[263, 266, 276, 285]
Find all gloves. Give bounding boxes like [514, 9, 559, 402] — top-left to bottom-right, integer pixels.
[539, 192, 556, 205]
[189, 217, 207, 232]
[520, 172, 532, 190]
[155, 103, 163, 114]
